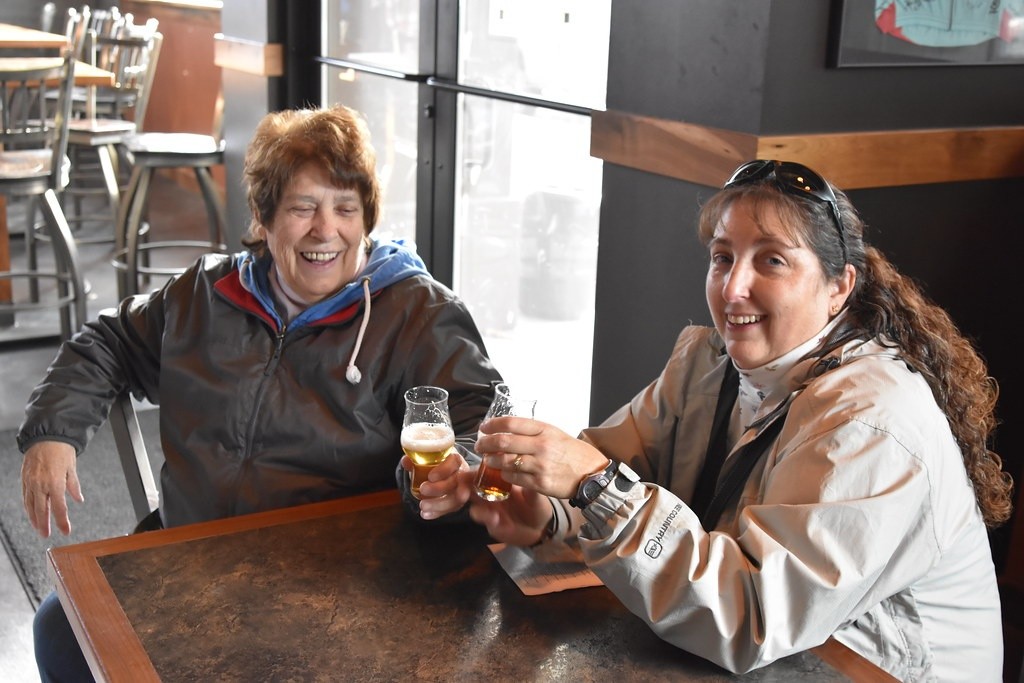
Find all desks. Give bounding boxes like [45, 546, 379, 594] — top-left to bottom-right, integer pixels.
[0, 24, 71, 328]
[2, 56, 113, 87]
[46, 489, 902, 683]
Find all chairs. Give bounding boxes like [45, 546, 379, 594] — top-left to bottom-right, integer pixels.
[0, 6, 224, 348]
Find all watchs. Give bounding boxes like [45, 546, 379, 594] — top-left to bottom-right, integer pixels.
[569, 459, 618, 511]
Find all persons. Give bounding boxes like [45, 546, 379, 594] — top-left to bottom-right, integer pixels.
[469, 158, 1015, 683]
[14, 106, 510, 683]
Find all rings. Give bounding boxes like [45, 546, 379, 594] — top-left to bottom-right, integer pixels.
[513, 454, 524, 472]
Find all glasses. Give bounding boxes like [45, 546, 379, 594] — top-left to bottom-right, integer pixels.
[723, 159, 847, 262]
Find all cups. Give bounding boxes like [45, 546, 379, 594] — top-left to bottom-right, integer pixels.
[477, 383, 538, 500]
[397, 387, 456, 497]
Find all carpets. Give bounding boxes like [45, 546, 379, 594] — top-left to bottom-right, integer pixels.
[1, 407, 167, 611]
